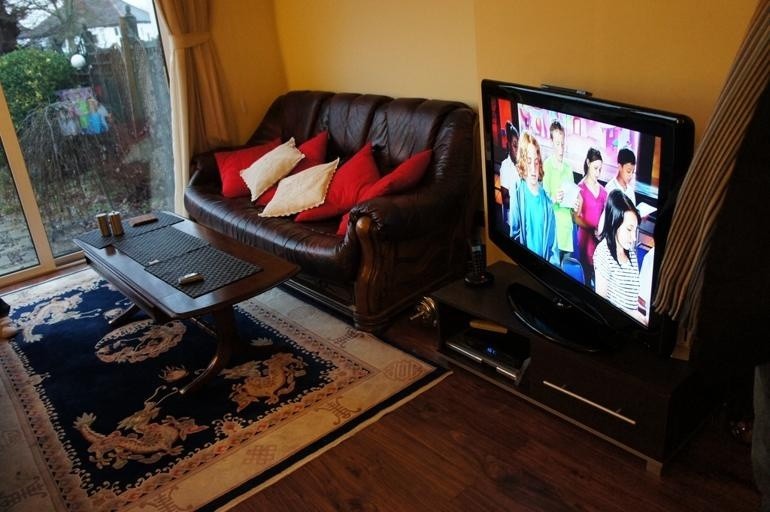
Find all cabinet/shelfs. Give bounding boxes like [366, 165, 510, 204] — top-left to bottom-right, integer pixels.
[430, 261, 722, 475]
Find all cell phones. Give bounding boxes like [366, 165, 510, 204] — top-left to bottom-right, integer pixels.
[178, 270, 202, 288]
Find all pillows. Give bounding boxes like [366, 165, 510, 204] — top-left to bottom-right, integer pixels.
[213, 130, 433, 237]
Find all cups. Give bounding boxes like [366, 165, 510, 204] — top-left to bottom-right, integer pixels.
[107, 212, 123, 237]
[96, 213, 112, 238]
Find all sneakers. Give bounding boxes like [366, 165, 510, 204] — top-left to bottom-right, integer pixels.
[0, 316, 18, 339]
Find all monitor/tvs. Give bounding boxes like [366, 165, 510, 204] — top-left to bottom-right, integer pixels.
[480, 79, 695, 357]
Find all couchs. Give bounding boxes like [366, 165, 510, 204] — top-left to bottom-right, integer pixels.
[183, 90, 476, 335]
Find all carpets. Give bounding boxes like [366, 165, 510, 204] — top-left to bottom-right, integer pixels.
[0, 253, 454, 512]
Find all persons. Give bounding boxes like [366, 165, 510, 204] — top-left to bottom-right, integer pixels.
[51, 91, 111, 169]
[496, 105, 655, 324]
